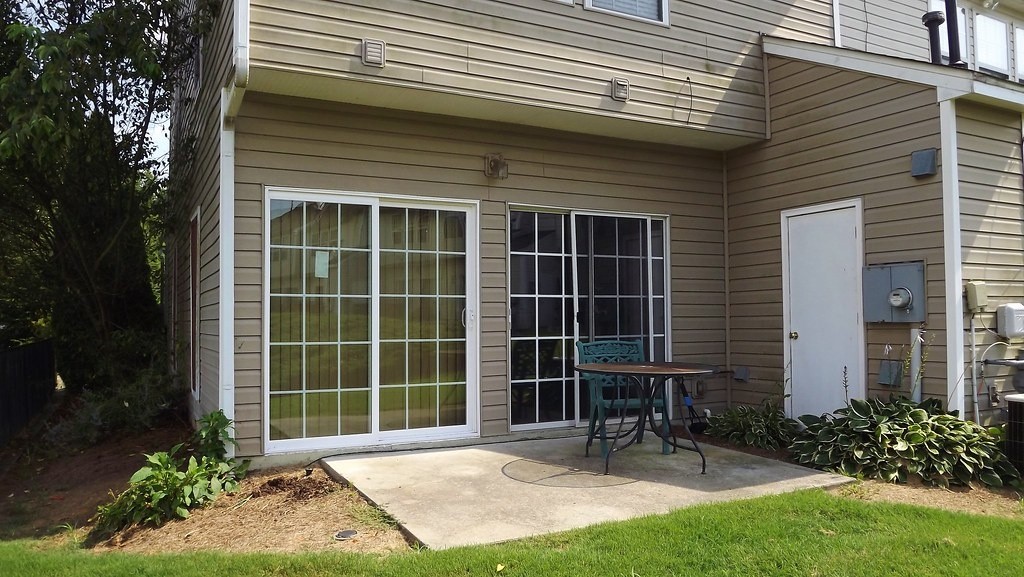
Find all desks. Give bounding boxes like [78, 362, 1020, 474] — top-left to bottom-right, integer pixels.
[575, 361, 720, 475]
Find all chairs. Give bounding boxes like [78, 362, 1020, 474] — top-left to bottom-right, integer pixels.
[575, 340, 670, 457]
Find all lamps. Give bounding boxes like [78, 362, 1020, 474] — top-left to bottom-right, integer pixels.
[484, 153, 510, 180]
[983, 0, 999, 10]
